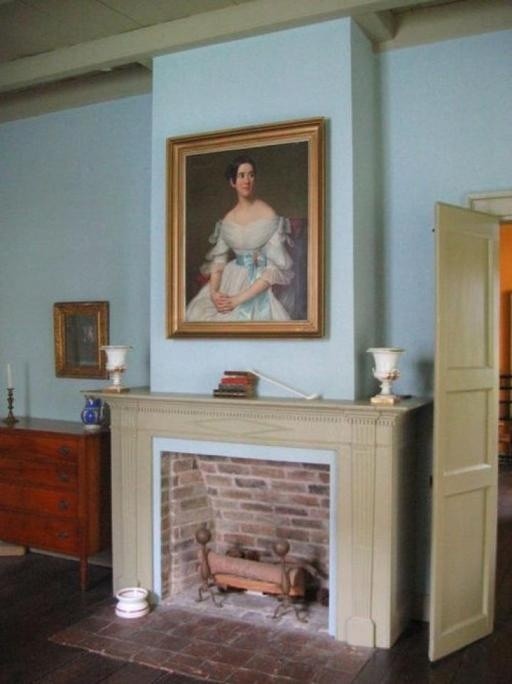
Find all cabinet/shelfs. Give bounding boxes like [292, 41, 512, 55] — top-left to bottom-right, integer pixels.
[0, 417, 111, 591]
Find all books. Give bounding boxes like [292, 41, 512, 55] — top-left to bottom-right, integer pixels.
[213, 370, 258, 399]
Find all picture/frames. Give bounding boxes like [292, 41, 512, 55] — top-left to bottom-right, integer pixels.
[53, 301, 110, 380]
[165, 117, 326, 338]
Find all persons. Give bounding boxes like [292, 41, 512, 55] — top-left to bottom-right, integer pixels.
[184, 155, 294, 321]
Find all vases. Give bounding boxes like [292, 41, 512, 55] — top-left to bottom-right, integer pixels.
[79, 389, 110, 430]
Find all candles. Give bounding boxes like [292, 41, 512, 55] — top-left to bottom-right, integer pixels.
[2, 363, 19, 424]
